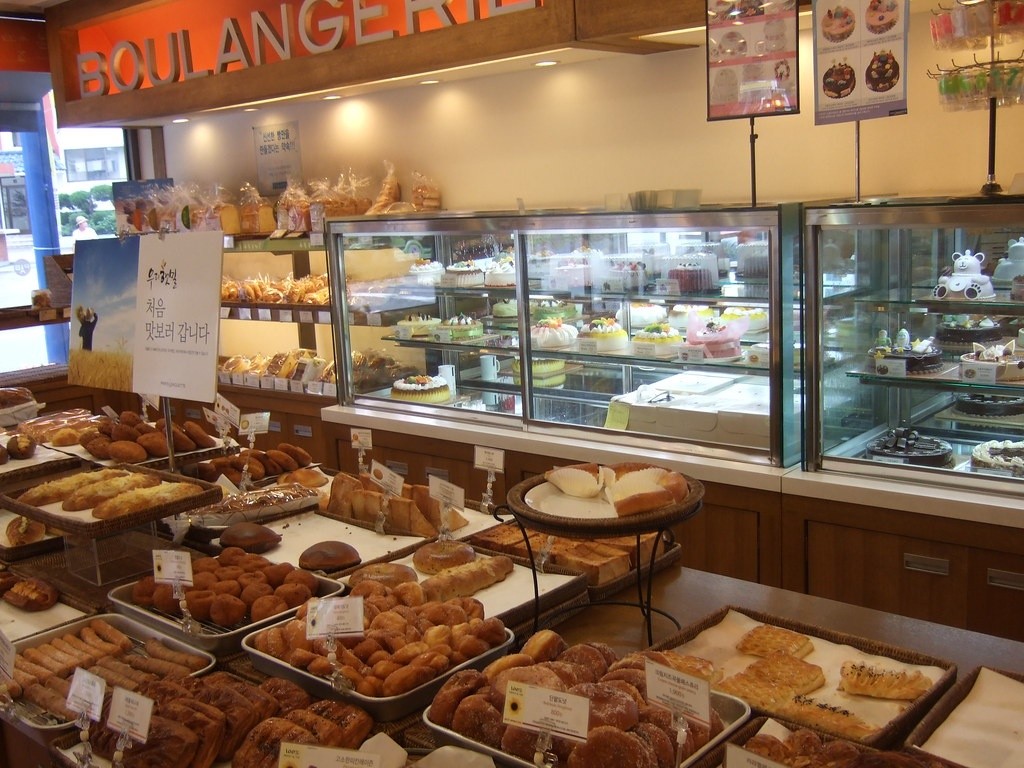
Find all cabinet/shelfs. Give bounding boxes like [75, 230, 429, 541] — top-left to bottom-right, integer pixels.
[218, 233, 440, 398]
[798, 193, 1024, 497]
[324, 204, 879, 468]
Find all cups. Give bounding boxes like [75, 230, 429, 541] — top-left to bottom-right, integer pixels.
[438, 365, 456, 390]
[480, 355, 500, 380]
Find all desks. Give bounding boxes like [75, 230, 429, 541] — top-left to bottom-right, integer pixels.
[0, 566, 1024, 768]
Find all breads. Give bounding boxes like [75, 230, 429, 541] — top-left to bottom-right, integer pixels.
[185, 442, 330, 524]
[427, 623, 950, 768]
[220, 347, 419, 392]
[0, 385, 216, 548]
[221, 273, 332, 306]
[324, 470, 469, 537]
[544, 461, 688, 516]
[148, 181, 440, 234]
[0, 525, 666, 768]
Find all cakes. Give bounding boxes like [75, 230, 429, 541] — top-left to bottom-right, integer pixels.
[709, 0, 793, 104]
[492, 299, 577, 321]
[613, 301, 771, 330]
[408, 256, 517, 287]
[865, 426, 954, 470]
[570, 245, 772, 274]
[526, 314, 802, 367]
[970, 439, 1024, 478]
[396, 311, 484, 342]
[552, 260, 719, 293]
[958, 339, 1024, 381]
[865, 0, 899, 34]
[513, 374, 566, 387]
[934, 315, 1024, 346]
[821, 5, 856, 42]
[866, 49, 899, 92]
[511, 357, 565, 373]
[823, 63, 856, 99]
[391, 375, 450, 403]
[865, 328, 944, 375]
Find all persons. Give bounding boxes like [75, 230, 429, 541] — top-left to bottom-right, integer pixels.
[79, 307, 98, 351]
[72, 216, 99, 253]
[492, 239, 515, 264]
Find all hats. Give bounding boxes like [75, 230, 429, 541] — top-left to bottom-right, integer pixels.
[75, 215, 89, 225]
